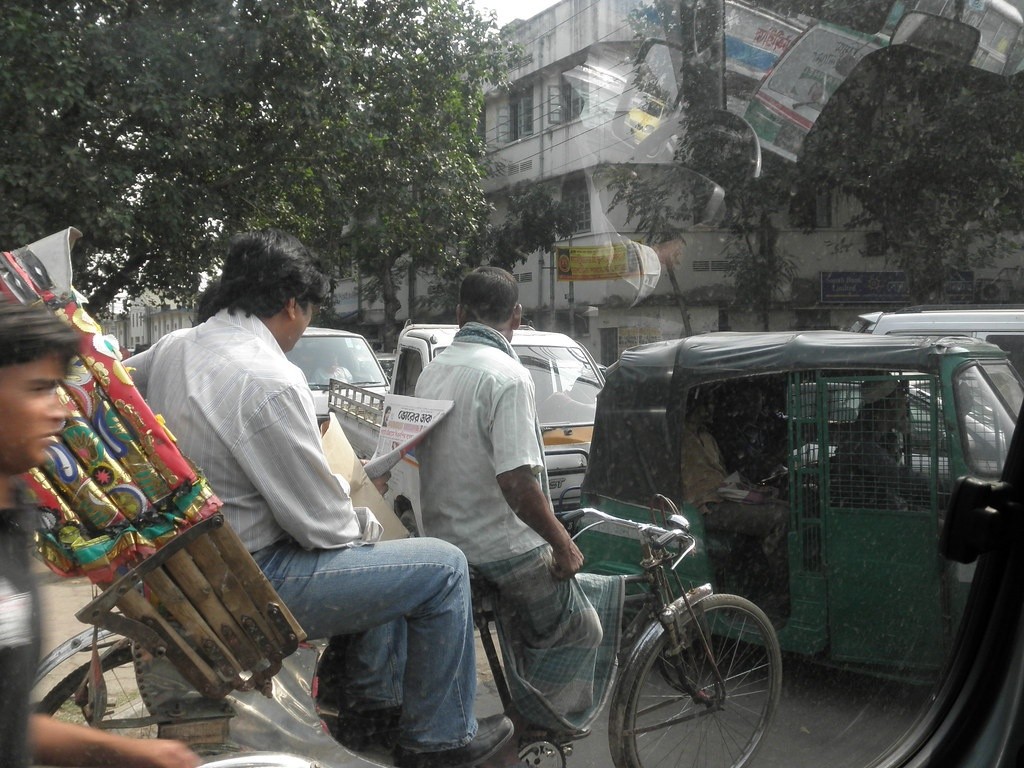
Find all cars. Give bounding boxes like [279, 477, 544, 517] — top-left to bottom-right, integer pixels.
[787, 382, 1012, 496]
[289, 328, 391, 463]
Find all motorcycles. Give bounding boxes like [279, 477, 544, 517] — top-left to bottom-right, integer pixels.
[577, 334, 1019, 694]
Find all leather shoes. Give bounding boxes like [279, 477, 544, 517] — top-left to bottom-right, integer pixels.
[320, 705, 403, 757]
[395, 715, 515, 768]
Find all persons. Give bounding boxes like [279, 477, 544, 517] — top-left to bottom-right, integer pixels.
[827, 377, 942, 511]
[414, 267, 592, 741]
[678, 376, 793, 616]
[0, 305, 202, 768]
[120, 227, 515, 768]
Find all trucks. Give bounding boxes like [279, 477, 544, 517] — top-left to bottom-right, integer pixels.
[325, 317, 606, 537]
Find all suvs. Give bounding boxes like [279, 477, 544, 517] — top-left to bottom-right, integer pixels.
[857, 305, 1024, 413]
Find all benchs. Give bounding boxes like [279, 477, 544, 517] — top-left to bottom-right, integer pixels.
[703, 532, 763, 572]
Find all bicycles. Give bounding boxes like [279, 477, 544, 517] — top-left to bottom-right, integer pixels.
[25, 494, 784, 768]
